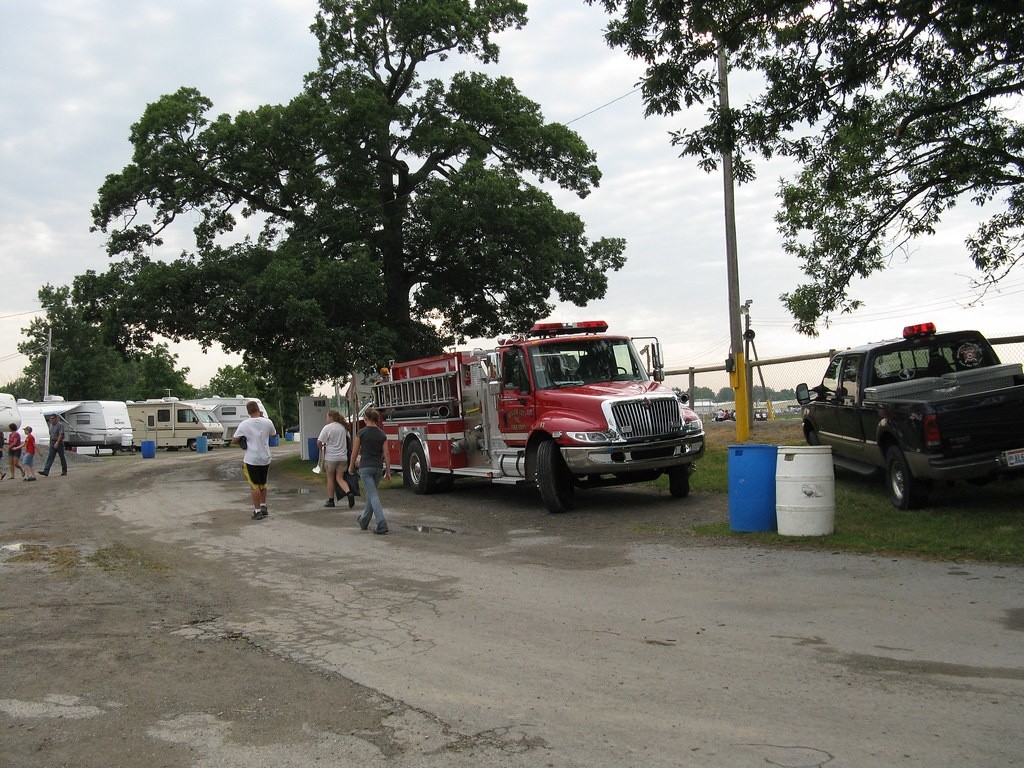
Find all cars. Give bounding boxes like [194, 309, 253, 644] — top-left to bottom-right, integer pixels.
[345, 401, 374, 423]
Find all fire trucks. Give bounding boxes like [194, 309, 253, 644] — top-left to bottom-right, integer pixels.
[369, 320, 706, 515]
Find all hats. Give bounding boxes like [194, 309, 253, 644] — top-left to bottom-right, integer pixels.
[23, 426, 32, 432]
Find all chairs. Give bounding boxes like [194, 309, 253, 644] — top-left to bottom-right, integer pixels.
[574, 355, 593, 379]
[928, 354, 955, 377]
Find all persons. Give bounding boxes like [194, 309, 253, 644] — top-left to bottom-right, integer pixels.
[348, 408, 392, 534]
[37, 414, 67, 476]
[232, 401, 276, 519]
[715, 409, 764, 420]
[317, 410, 355, 508]
[0, 423, 43, 480]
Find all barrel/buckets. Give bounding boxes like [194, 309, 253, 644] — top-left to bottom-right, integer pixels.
[294, 433, 300, 442]
[728, 444, 777, 531]
[285, 433, 293, 441]
[270, 435, 279, 446]
[141, 441, 155, 457]
[776, 446, 835, 536]
[196, 436, 208, 453]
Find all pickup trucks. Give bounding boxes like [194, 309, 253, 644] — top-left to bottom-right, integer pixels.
[795, 323, 1024, 512]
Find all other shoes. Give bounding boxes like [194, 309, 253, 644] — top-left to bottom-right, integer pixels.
[22, 474, 36, 481]
[8, 477, 15, 479]
[260, 506, 268, 515]
[323, 500, 335, 507]
[38, 471, 48, 476]
[347, 493, 355, 507]
[356, 514, 368, 530]
[0, 473, 6, 480]
[251, 510, 264, 520]
[372, 527, 390, 534]
[21, 468, 25, 477]
[60, 472, 67, 476]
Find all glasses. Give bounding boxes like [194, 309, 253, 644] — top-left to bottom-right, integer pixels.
[49, 418, 54, 419]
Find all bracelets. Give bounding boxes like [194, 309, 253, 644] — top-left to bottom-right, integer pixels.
[56, 441, 59, 443]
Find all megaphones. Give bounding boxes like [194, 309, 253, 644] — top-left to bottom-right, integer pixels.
[744, 330, 755, 341]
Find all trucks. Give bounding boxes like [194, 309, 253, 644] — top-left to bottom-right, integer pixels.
[179, 393, 270, 448]
[125, 396, 225, 450]
